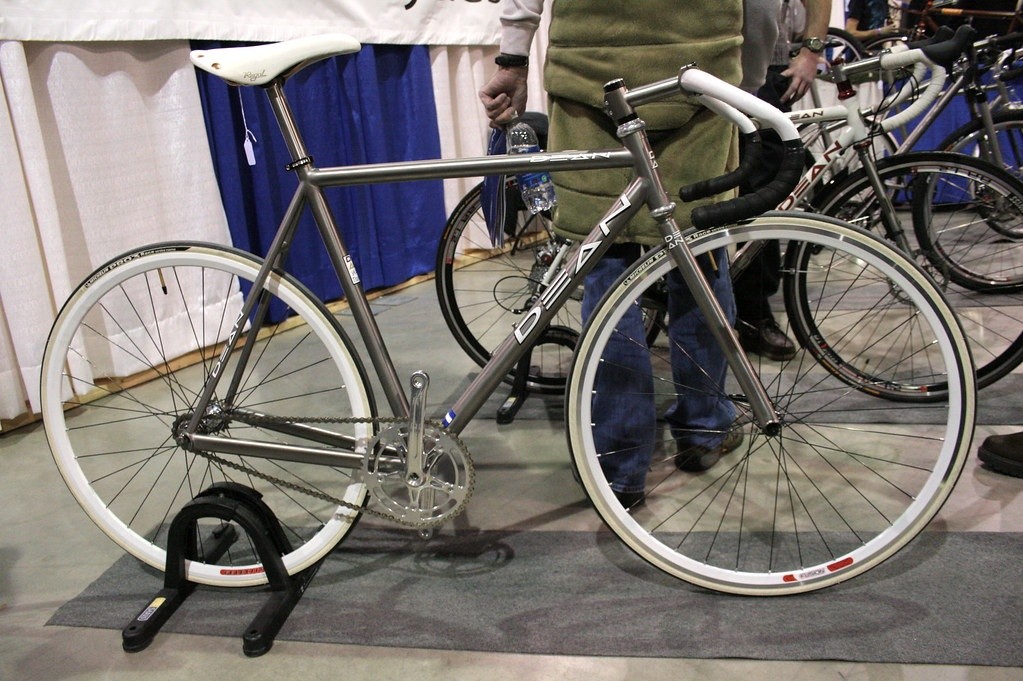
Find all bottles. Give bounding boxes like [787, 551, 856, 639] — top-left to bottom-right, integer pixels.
[505, 106, 556, 212]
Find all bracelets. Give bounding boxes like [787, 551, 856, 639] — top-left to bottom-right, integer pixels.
[877, 27, 884, 37]
[494, 51, 529, 69]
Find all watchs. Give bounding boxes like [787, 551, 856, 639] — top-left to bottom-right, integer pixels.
[799, 36, 827, 53]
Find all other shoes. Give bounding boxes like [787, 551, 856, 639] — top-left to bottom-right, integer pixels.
[571, 460, 647, 507]
[977, 430, 1023, 476]
[734, 318, 797, 361]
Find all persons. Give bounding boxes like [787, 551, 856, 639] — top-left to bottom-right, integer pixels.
[475, 0, 782, 519]
[843, 0, 896, 154]
[737, 0, 832, 363]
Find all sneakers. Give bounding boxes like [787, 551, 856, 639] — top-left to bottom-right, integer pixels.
[675, 418, 746, 472]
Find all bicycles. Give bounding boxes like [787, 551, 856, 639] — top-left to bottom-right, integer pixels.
[433, 34, 1023, 401]
[759, 9, 1023, 291]
[37, 29, 978, 601]
